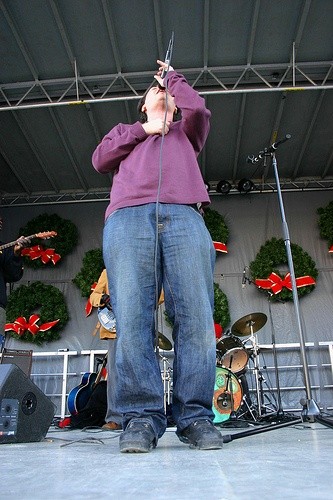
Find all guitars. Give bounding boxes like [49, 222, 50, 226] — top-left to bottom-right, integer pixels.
[97, 305, 118, 332]
[0, 230, 58, 250]
[67, 351, 108, 416]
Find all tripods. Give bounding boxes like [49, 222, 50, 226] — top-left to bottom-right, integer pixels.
[222, 133, 333, 443]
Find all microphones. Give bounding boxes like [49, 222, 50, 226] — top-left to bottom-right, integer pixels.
[241, 268, 246, 288]
[158, 38, 174, 90]
[222, 390, 228, 407]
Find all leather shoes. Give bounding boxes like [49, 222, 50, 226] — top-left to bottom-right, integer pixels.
[101, 422, 122, 430]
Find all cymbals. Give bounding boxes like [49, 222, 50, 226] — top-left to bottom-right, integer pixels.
[232, 311, 268, 337]
[154, 329, 173, 350]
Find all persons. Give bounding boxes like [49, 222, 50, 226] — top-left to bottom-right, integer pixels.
[92, 59, 226, 453]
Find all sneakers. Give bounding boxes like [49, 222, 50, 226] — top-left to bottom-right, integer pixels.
[179, 419, 223, 450]
[119, 422, 156, 452]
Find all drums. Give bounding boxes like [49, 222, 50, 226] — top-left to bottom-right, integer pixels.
[216, 334, 250, 373]
[211, 365, 244, 424]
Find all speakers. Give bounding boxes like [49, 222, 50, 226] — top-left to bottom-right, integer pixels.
[1, 348, 33, 376]
[0, 363, 57, 445]
